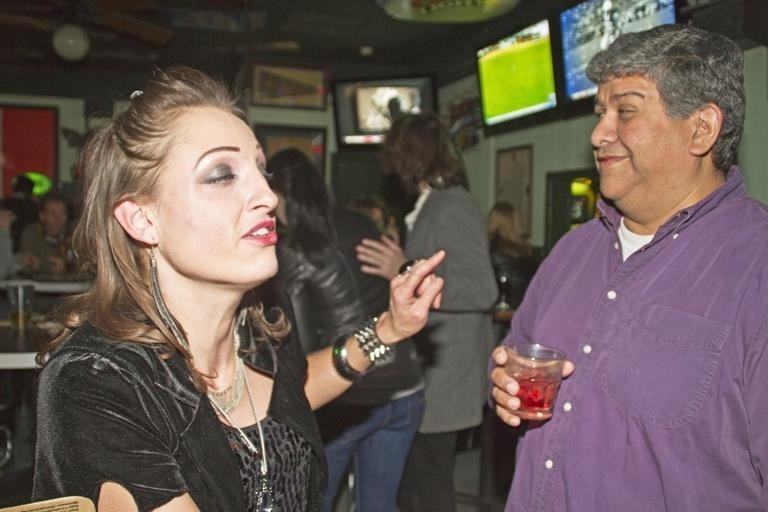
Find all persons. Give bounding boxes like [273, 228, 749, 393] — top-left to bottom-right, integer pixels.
[32, 60, 450, 511]
[474, 13, 768, 510]
[1, 90, 530, 510]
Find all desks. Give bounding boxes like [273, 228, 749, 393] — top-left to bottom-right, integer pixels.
[0, 276, 84, 370]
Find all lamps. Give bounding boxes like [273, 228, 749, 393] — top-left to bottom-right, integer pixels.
[52, 23, 90, 63]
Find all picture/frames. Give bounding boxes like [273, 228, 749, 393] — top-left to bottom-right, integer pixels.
[250, 59, 329, 111]
[254, 125, 326, 183]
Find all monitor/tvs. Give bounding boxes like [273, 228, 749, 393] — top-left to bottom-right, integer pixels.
[332, 74, 438, 149]
[555, 0, 682, 119]
[470, 12, 562, 138]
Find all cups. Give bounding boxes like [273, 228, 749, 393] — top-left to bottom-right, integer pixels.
[504, 341, 566, 422]
